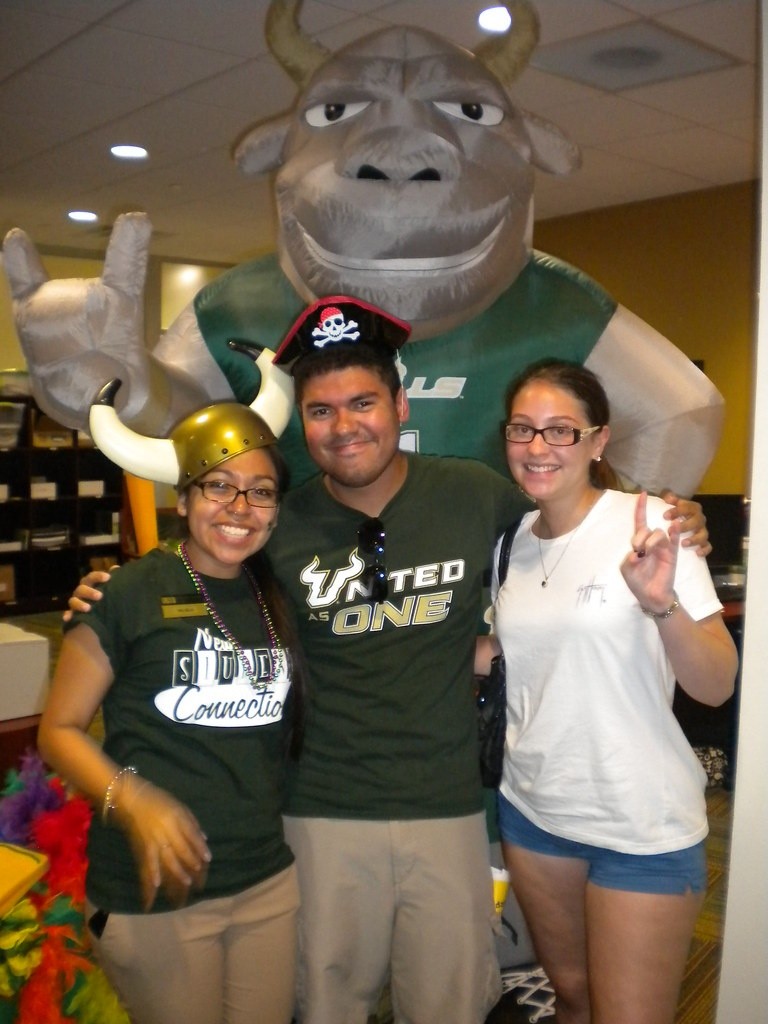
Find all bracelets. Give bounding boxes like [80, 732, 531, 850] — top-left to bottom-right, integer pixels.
[106, 767, 137, 808]
[641, 592, 679, 618]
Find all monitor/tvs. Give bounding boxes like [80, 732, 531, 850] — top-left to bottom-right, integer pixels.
[688, 494, 745, 565]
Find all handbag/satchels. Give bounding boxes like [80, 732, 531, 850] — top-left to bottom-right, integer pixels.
[470, 517, 523, 789]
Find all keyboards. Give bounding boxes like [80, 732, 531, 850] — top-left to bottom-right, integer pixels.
[715, 585, 745, 601]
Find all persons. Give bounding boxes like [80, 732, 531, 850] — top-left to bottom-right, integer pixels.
[39, 404, 309, 1023]
[474, 361, 738, 1024]
[62, 295, 714, 1024]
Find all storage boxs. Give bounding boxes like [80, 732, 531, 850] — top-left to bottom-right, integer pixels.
[0, 622, 53, 722]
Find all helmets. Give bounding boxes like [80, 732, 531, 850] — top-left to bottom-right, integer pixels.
[170, 402, 279, 490]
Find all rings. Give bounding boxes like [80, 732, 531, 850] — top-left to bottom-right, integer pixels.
[162, 844, 171, 848]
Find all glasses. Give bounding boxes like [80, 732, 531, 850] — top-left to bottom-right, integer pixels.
[358, 515, 389, 606]
[504, 419, 602, 446]
[195, 479, 280, 508]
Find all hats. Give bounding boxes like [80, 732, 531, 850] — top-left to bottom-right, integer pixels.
[272, 296, 412, 371]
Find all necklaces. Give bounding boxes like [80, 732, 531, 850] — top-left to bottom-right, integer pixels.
[177, 541, 283, 690]
[538, 525, 581, 588]
